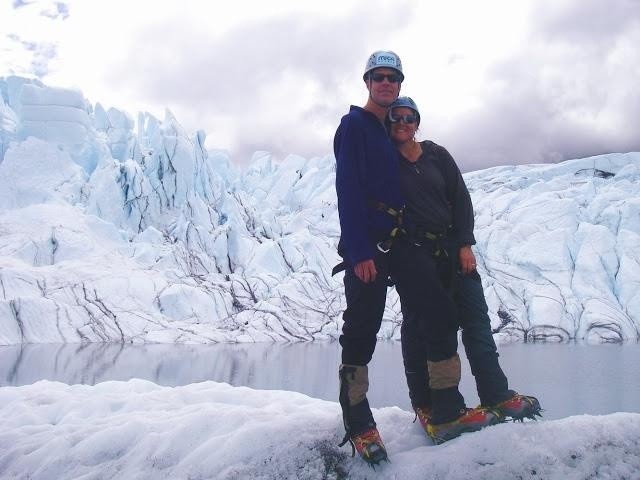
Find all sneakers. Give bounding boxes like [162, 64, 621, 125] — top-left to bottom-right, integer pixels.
[415, 405, 498, 439]
[344, 420, 386, 464]
[481, 394, 540, 419]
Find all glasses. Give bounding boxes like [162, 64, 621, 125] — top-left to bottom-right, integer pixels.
[389, 114, 417, 123]
[366, 73, 400, 83]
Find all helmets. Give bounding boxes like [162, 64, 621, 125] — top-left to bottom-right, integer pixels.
[363, 50, 404, 82]
[390, 96, 420, 116]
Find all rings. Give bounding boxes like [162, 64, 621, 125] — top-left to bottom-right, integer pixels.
[472, 263, 475, 266]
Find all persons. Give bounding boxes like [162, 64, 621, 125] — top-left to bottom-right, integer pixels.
[384, 96, 535, 447]
[332, 50, 406, 467]
